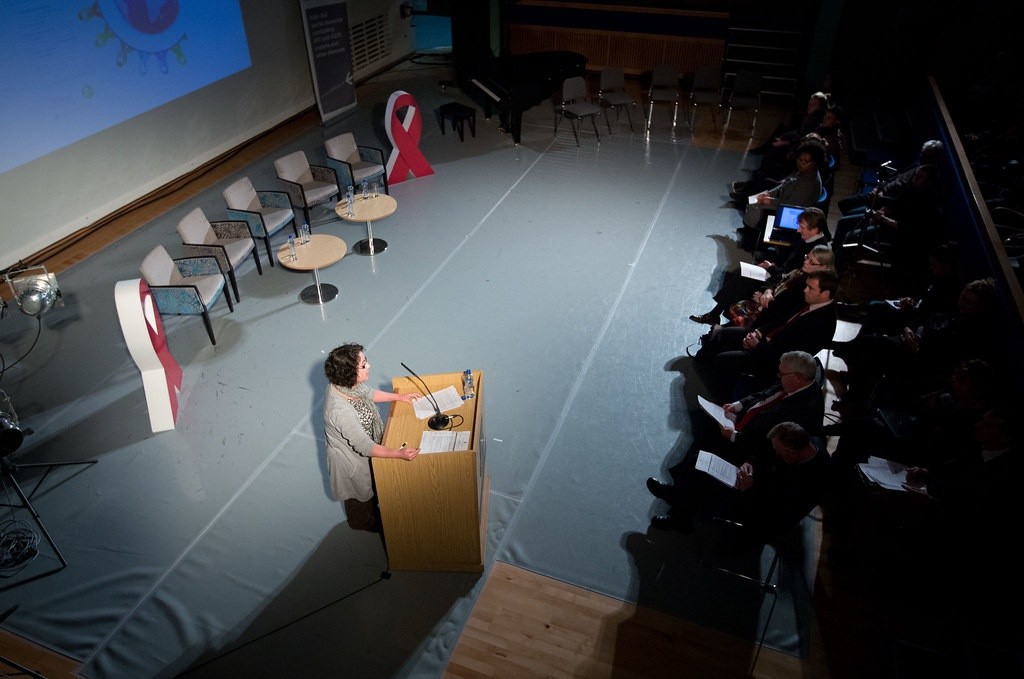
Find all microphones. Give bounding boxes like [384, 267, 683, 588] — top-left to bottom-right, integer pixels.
[400, 362, 449, 429]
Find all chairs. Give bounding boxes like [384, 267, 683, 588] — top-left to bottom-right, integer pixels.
[175, 207, 263, 303]
[599, 67, 636, 137]
[655, 145, 835, 604]
[138, 245, 235, 346]
[724, 70, 763, 132]
[222, 175, 300, 269]
[689, 66, 723, 133]
[555, 75, 602, 149]
[323, 132, 390, 198]
[273, 149, 344, 237]
[647, 66, 679, 129]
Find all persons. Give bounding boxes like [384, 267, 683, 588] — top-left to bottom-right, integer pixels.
[324, 345, 421, 533]
[646, 72, 1024, 554]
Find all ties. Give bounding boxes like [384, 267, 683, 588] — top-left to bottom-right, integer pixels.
[735, 392, 788, 431]
[768, 305, 811, 339]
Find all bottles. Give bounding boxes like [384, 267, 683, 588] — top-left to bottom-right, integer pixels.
[347, 183, 354, 202]
[463, 368, 475, 398]
[346, 195, 353, 209]
[302, 221, 311, 241]
[288, 234, 296, 258]
[937, 393, 953, 414]
[361, 180, 370, 197]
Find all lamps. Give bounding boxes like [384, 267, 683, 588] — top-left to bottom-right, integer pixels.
[6, 265, 64, 315]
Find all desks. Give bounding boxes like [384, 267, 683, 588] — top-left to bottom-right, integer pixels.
[334, 194, 397, 257]
[763, 215, 793, 247]
[276, 233, 346, 305]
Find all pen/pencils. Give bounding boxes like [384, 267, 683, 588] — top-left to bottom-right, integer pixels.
[399, 442, 407, 450]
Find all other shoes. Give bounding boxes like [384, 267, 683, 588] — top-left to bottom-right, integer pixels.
[737, 228, 745, 234]
[646, 477, 683, 509]
[687, 345, 703, 358]
[836, 302, 856, 313]
[824, 369, 843, 381]
[730, 192, 746, 202]
[651, 515, 673, 531]
[748, 148, 764, 156]
[689, 313, 721, 326]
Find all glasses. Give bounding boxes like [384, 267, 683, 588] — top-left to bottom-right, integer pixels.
[359, 356, 367, 369]
[777, 370, 796, 380]
[804, 254, 823, 265]
[797, 157, 813, 165]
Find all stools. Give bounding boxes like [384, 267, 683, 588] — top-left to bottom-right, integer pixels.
[436, 100, 477, 141]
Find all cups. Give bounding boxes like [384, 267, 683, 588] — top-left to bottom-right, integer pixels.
[298, 228, 306, 245]
[348, 203, 353, 216]
[373, 183, 380, 196]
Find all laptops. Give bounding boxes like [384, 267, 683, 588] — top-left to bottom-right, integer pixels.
[770, 204, 807, 244]
